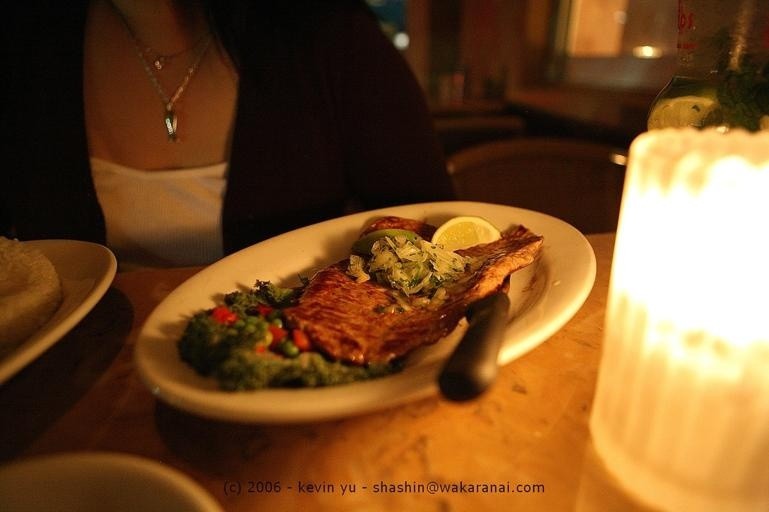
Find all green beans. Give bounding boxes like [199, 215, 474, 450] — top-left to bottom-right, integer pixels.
[226, 318, 300, 358]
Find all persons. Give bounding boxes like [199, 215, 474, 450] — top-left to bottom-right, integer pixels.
[1, 1, 459, 269]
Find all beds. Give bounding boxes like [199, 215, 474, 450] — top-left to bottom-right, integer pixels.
[2, 231, 769, 512]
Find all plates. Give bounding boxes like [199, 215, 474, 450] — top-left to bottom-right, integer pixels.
[2, 238, 119, 388]
[133, 199, 599, 426]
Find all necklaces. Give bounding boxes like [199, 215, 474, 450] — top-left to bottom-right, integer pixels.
[107, 0, 216, 142]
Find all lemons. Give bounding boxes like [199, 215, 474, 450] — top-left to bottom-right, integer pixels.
[432, 215, 502, 251]
